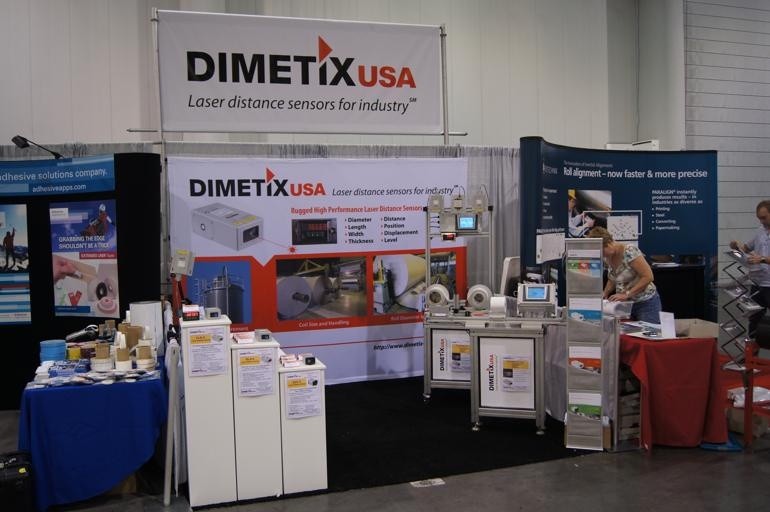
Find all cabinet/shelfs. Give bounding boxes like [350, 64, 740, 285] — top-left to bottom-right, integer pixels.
[279, 352, 329, 495]
[180, 314, 237, 507]
[232, 330, 283, 501]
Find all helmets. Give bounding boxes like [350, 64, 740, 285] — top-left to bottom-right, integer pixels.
[568, 189, 577, 200]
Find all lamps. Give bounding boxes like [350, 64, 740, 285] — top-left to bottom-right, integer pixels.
[13, 134, 66, 159]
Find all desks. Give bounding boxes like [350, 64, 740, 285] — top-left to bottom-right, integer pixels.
[620, 322, 729, 450]
[17, 342, 167, 512]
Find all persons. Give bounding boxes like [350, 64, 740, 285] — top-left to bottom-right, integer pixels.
[588, 226, 662, 324]
[731, 200, 770, 363]
[53, 253, 76, 283]
[89, 203, 114, 235]
[568, 189, 594, 238]
[2, 228, 17, 268]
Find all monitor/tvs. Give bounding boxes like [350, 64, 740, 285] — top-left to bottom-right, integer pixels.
[457, 212, 476, 229]
[524, 285, 548, 301]
